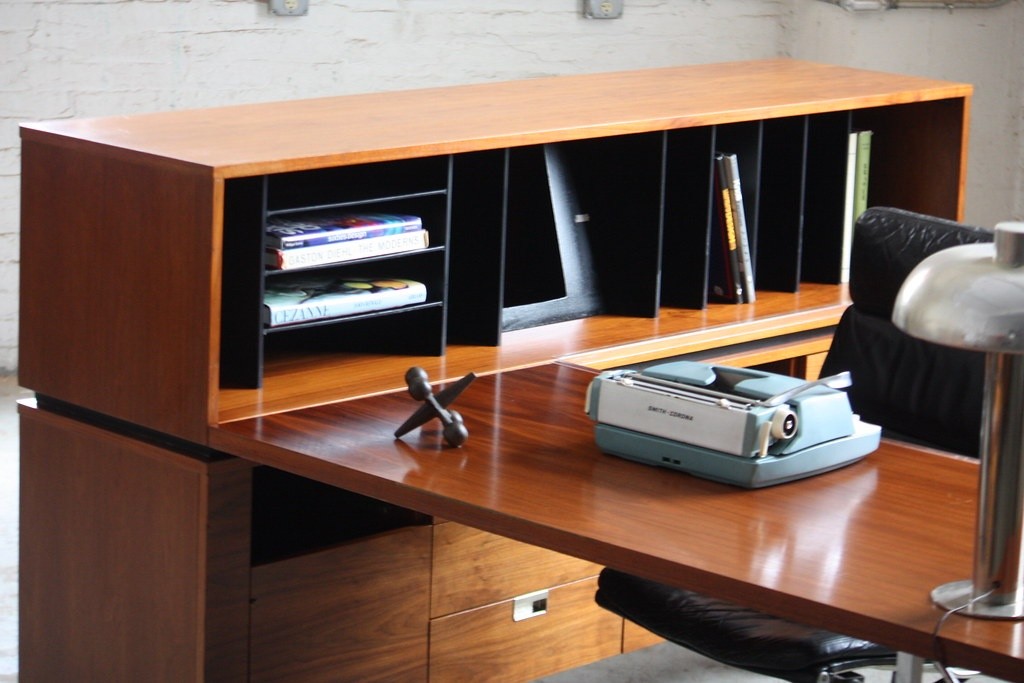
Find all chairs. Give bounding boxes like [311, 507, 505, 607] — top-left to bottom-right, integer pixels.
[595, 207, 998, 683]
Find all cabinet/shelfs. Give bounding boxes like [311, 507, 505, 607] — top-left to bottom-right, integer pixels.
[17, 51, 974, 683]
[221, 155, 458, 392]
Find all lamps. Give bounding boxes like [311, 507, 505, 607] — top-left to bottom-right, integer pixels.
[890, 221, 1023, 621]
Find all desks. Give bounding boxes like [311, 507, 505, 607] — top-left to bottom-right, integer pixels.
[205, 359, 1024, 683]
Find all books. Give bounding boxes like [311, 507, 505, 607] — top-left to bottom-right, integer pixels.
[713, 152, 757, 305]
[259, 213, 431, 328]
[842, 130, 871, 281]
